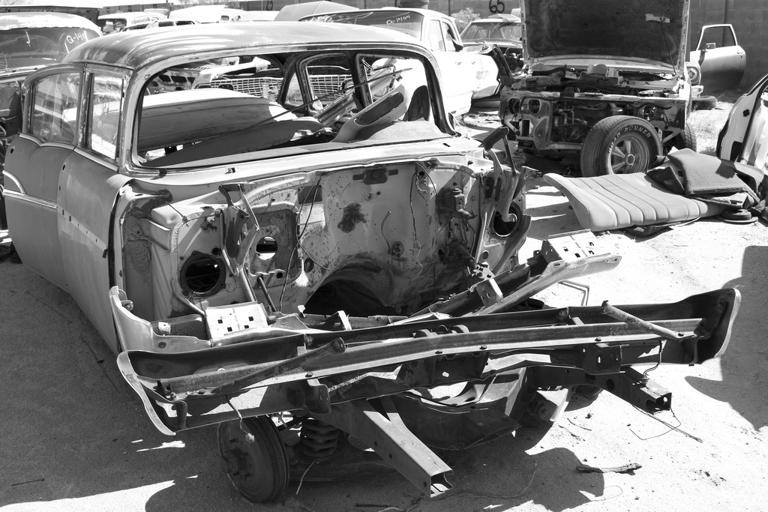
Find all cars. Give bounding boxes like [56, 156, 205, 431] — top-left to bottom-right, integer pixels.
[0, 19, 744, 507]
[0, 0, 767, 178]
